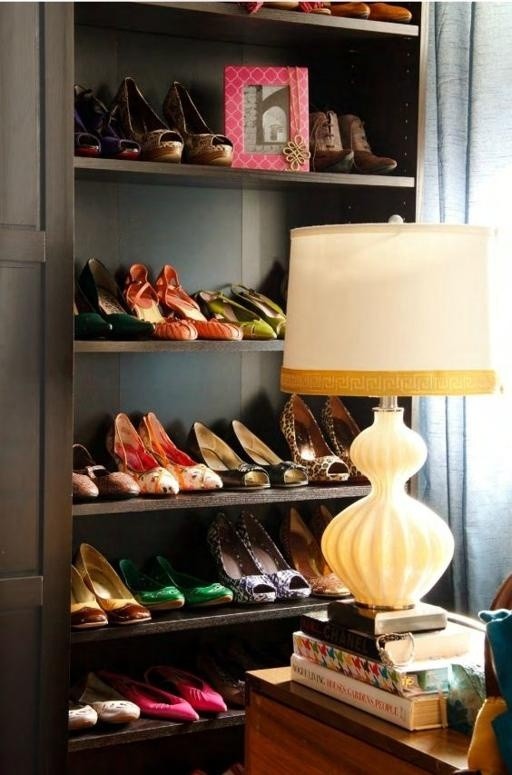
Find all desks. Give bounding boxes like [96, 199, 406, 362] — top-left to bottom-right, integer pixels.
[242, 663, 512, 775]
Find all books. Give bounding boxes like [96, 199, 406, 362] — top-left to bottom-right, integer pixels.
[326, 600, 448, 635]
[289, 651, 452, 732]
[298, 611, 465, 667]
[291, 629, 450, 699]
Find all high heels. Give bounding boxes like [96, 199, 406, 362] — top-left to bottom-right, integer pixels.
[279, 393, 350, 483]
[104, 409, 186, 499]
[70, 469, 101, 504]
[170, 76, 235, 166]
[317, 390, 369, 484]
[194, 289, 278, 339]
[200, 510, 281, 615]
[83, 86, 143, 160]
[341, 105, 397, 172]
[308, 104, 352, 173]
[136, 410, 226, 494]
[154, 262, 243, 342]
[221, 417, 311, 487]
[115, 554, 188, 613]
[158, 547, 233, 609]
[229, 280, 288, 337]
[73, 84, 102, 159]
[278, 507, 369, 599]
[57, 561, 113, 632]
[75, 443, 139, 501]
[126, 256, 198, 342]
[71, 255, 112, 341]
[81, 257, 156, 337]
[187, 414, 274, 491]
[78, 536, 153, 632]
[122, 76, 185, 162]
[237, 510, 314, 604]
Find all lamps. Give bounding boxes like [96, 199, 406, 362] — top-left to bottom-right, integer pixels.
[277, 212, 498, 640]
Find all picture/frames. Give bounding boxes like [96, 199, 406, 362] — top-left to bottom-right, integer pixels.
[223, 63, 312, 173]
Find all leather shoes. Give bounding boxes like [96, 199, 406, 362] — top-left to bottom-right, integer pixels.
[147, 659, 230, 721]
[199, 650, 250, 710]
[102, 668, 203, 726]
[65, 698, 98, 728]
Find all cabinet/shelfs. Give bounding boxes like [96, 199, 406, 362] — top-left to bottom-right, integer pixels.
[45, 0, 435, 775]
